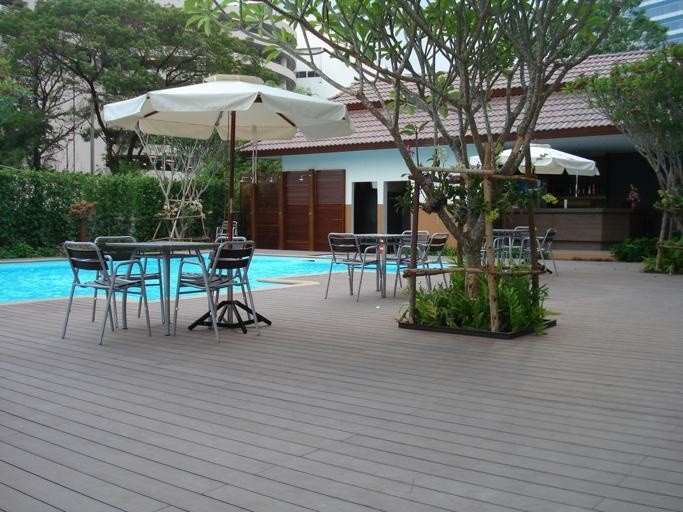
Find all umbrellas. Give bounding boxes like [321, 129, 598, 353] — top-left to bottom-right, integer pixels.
[469, 139, 600, 274]
[104, 66, 357, 335]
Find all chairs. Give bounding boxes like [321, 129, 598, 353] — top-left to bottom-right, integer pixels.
[90, 236, 166, 329]
[324, 232, 379, 303]
[173, 240, 260, 342]
[181, 236, 246, 328]
[216, 220, 238, 240]
[62, 241, 151, 346]
[393, 233, 450, 296]
[479, 224, 558, 276]
[386, 230, 430, 289]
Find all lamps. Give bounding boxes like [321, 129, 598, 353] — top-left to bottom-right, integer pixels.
[298, 174, 309, 181]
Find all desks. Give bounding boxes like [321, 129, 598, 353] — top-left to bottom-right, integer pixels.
[355, 233, 411, 298]
[105, 241, 219, 336]
